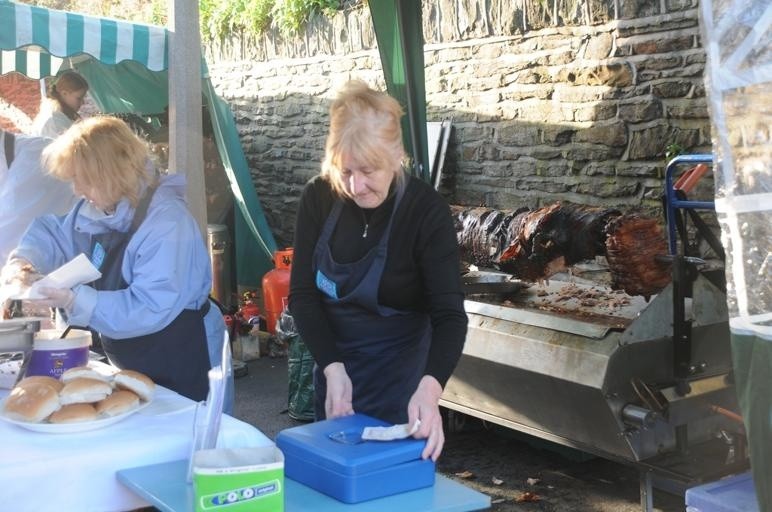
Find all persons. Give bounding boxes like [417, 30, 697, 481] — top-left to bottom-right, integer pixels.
[29, 71, 89, 141]
[288, 81, 469, 461]
[2, 115, 234, 413]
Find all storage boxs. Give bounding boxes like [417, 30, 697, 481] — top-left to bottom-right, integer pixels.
[684, 470, 756, 512]
[192, 447, 285, 512]
[275, 412, 435, 504]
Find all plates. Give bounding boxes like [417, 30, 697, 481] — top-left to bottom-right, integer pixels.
[1, 396, 152, 435]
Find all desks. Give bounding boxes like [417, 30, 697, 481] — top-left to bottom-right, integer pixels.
[0, 384, 276, 512]
[116, 457, 491, 512]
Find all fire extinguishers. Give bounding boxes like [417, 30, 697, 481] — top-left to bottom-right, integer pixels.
[239, 289, 260, 334]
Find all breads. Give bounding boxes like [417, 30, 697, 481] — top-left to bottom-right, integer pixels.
[15, 376, 64, 393]
[95, 390, 140, 419]
[6, 383, 60, 423]
[114, 369, 155, 402]
[48, 403, 96, 424]
[60, 365, 103, 382]
[58, 378, 113, 406]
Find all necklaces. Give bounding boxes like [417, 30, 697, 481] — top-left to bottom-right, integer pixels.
[358, 207, 380, 238]
